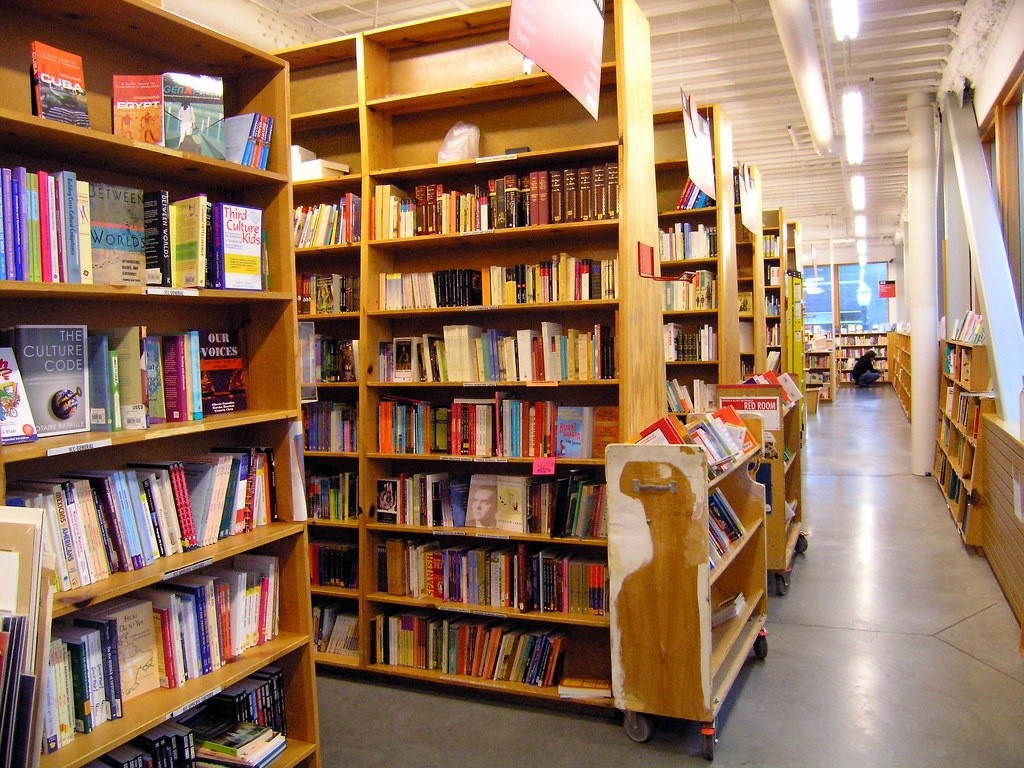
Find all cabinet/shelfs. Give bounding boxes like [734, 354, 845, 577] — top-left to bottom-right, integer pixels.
[1, 0, 1024, 768]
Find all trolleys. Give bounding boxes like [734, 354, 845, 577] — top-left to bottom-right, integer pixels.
[714, 372, 809, 597]
[605, 411, 768, 762]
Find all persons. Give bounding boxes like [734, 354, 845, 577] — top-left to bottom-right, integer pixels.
[852, 350, 884, 388]
[466, 485, 497, 528]
[398, 345, 408, 370]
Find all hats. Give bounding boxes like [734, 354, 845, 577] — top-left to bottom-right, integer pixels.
[181, 98, 190, 107]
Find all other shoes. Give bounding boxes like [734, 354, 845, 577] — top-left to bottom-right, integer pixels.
[860, 385, 870, 388]
[854, 381, 861, 385]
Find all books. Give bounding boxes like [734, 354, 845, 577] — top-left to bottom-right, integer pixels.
[0, 35, 983, 768]
